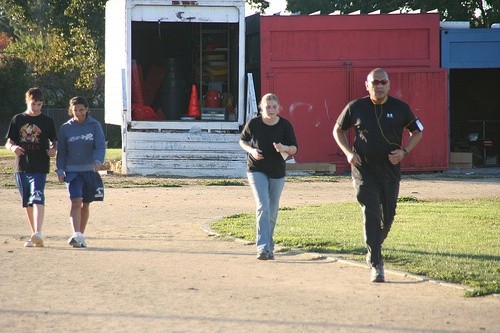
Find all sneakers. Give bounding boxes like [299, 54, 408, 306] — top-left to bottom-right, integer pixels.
[81, 237, 87, 247]
[69, 235, 82, 247]
[31, 232, 44, 247]
[25, 239, 34, 247]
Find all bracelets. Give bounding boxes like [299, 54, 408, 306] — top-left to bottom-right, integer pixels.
[403, 149, 407, 156]
[10, 144, 15, 150]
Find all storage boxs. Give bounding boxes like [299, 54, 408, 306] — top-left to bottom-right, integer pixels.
[449, 152, 473, 169]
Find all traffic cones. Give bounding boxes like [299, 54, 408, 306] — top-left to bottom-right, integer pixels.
[186, 85, 200, 116]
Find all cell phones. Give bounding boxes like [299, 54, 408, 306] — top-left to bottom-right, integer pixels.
[416, 118, 424, 131]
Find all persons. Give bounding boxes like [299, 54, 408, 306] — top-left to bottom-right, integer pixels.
[4, 89, 58, 247]
[56, 96, 107, 248]
[332, 68, 424, 282]
[239, 93, 298, 260]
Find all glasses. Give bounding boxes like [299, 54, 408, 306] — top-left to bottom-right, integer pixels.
[368, 79, 389, 85]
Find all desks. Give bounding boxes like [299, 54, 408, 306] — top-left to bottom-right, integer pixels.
[464, 118, 498, 166]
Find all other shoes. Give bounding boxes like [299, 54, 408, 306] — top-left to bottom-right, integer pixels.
[370, 263, 385, 282]
[257, 251, 268, 260]
[268, 252, 274, 259]
